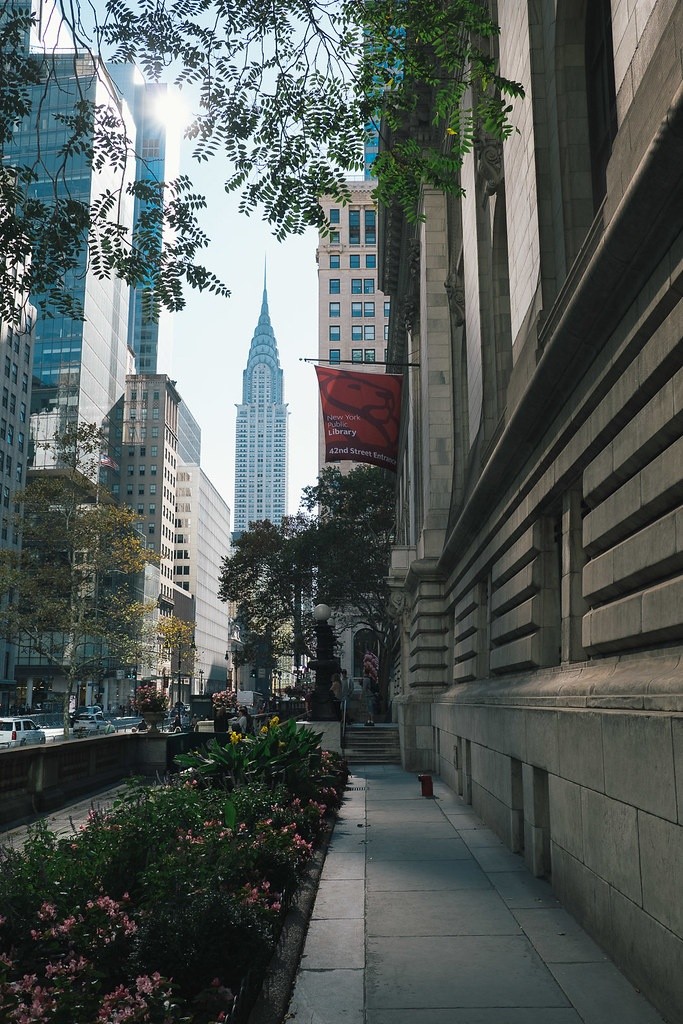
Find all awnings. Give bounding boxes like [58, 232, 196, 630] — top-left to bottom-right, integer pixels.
[1, 702, 31, 718]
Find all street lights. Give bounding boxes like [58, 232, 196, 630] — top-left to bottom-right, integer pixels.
[225, 646, 248, 695]
[176, 630, 199, 730]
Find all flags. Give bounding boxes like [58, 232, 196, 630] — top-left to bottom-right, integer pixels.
[99, 450, 119, 471]
[314, 364, 404, 474]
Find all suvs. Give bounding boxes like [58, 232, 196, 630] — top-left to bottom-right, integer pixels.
[72, 706, 103, 724]
[73, 714, 115, 735]
[1, 718, 46, 750]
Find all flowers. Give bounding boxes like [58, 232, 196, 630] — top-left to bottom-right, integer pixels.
[212, 687, 240, 709]
[0, 717, 351, 1024]
[131, 685, 169, 711]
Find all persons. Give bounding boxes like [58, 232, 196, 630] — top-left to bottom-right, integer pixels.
[119, 704, 144, 718]
[171, 714, 180, 731]
[330, 673, 343, 722]
[362, 668, 376, 726]
[168, 717, 175, 732]
[341, 669, 354, 723]
[232, 707, 247, 734]
[256, 706, 275, 725]
[104, 721, 115, 734]
[241, 706, 254, 735]
[137, 719, 147, 731]
